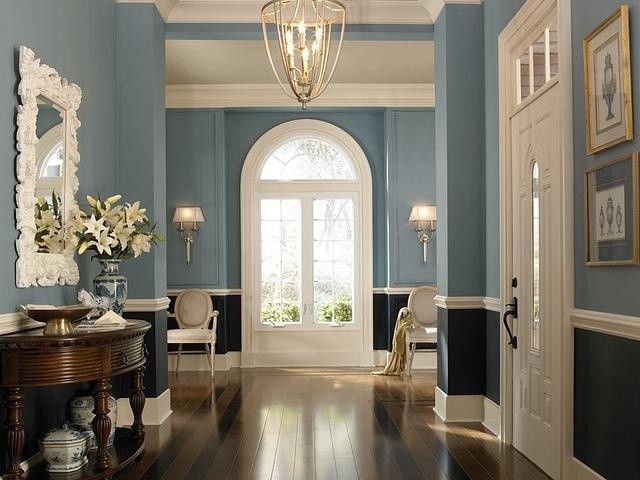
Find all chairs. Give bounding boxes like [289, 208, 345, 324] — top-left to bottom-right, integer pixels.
[167, 288, 219, 385]
[399, 285, 439, 378]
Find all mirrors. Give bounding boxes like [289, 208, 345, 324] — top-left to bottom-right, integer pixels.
[13, 41, 80, 292]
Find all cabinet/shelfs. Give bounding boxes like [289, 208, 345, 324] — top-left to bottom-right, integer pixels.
[0, 314, 153, 479]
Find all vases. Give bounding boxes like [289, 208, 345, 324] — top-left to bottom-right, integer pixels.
[91, 254, 128, 320]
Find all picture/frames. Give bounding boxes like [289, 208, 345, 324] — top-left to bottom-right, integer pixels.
[580, 5, 635, 156]
[581, 152, 640, 272]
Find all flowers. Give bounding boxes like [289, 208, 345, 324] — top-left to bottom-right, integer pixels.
[68, 190, 164, 262]
[32, 185, 64, 256]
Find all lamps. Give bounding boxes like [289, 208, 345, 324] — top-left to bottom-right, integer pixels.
[172, 206, 208, 265]
[260, 0, 346, 110]
[408, 204, 439, 265]
